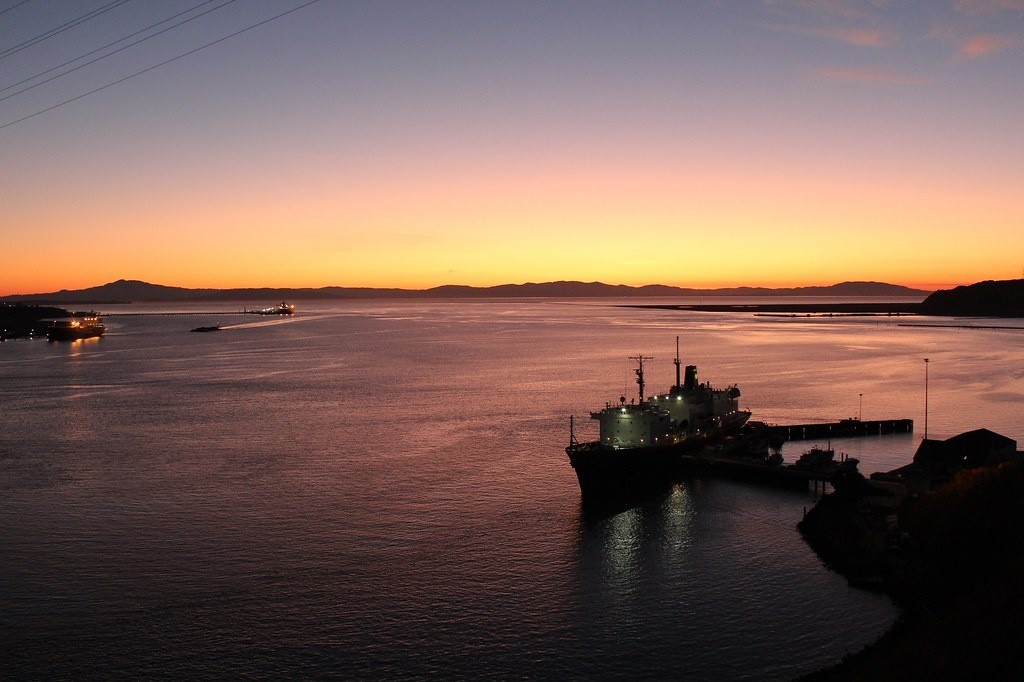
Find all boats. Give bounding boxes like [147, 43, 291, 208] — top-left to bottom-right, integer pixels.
[565, 333, 753, 502]
[278, 300, 294, 315]
[47, 315, 105, 343]
